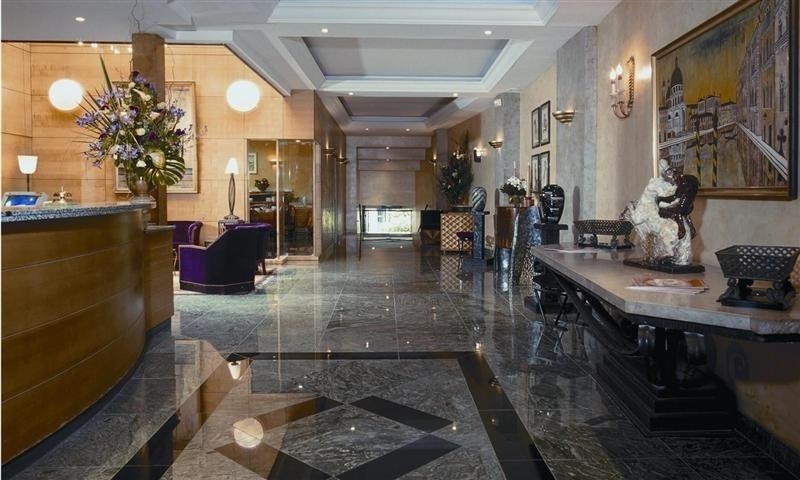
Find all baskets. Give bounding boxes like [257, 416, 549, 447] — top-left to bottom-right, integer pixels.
[715, 245, 799, 282]
[573, 220, 631, 235]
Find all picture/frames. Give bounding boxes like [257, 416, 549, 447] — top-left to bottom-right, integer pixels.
[248, 152, 258, 174]
[529, 101, 550, 193]
[651, 0, 799, 201]
[112, 80, 202, 195]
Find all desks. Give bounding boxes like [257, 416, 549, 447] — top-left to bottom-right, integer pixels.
[529, 241, 800, 438]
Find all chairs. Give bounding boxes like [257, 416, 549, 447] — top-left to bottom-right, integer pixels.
[167, 220, 273, 294]
[250, 196, 313, 244]
[529, 324, 593, 399]
[418, 210, 441, 250]
[532, 258, 568, 326]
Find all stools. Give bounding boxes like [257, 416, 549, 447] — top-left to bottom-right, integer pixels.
[457, 232, 474, 259]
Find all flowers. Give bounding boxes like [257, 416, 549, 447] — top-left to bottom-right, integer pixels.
[437, 128, 473, 208]
[71, 53, 208, 186]
[499, 175, 528, 203]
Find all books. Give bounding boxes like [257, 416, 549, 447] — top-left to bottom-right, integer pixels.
[624, 272, 712, 296]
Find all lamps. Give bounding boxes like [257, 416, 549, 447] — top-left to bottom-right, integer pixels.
[609, 55, 636, 120]
[224, 158, 240, 220]
[473, 147, 481, 162]
[552, 110, 575, 125]
[488, 140, 502, 149]
[17, 155, 39, 192]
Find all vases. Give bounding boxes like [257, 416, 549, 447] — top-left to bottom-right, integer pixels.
[508, 194, 527, 208]
[471, 187, 487, 211]
[537, 184, 565, 224]
[125, 175, 158, 202]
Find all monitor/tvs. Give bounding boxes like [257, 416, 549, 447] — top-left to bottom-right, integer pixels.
[1, 192, 49, 209]
